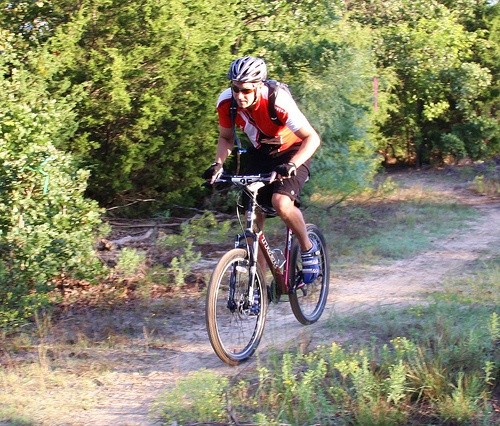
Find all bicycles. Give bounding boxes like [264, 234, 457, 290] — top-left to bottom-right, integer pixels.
[206, 169, 330, 366]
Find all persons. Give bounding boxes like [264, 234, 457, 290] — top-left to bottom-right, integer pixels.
[208, 55, 322, 314]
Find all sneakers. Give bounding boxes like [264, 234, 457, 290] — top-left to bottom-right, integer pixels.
[252, 290, 261, 314]
[301, 238, 320, 283]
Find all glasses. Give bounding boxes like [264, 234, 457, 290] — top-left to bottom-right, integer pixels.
[230, 86, 261, 95]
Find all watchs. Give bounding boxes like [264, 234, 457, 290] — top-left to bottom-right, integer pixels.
[287, 162, 296, 168]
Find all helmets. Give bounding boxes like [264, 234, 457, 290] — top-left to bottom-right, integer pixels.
[228, 56, 268, 83]
